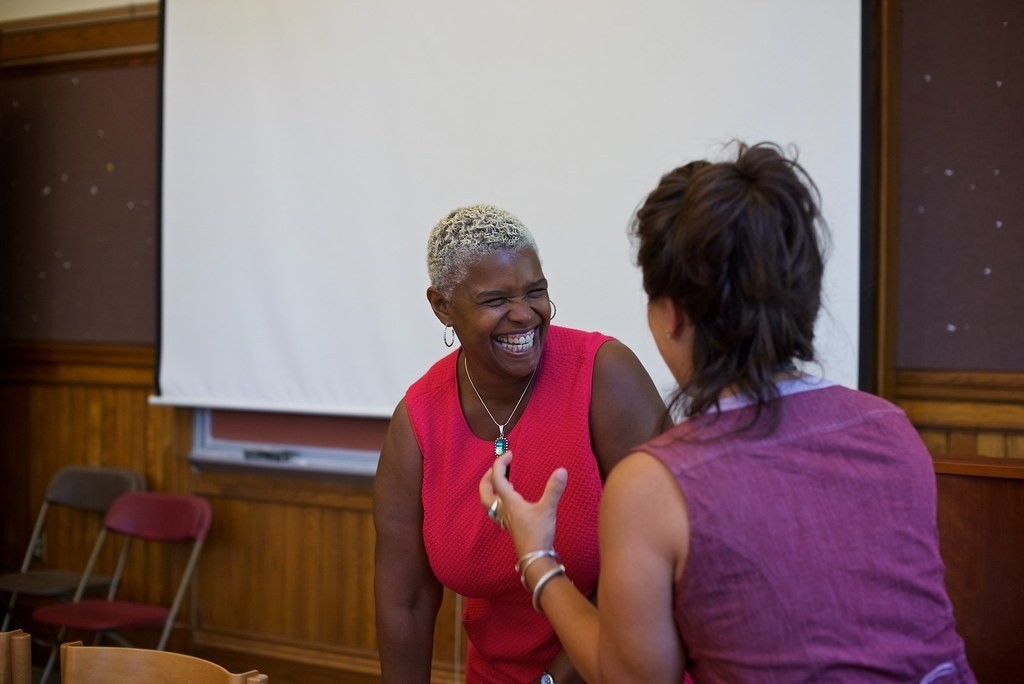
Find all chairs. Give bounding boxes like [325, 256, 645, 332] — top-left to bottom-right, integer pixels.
[0, 466, 146, 684]
[31, 493, 211, 684]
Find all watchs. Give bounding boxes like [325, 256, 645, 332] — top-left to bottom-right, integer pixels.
[540, 669, 556, 684]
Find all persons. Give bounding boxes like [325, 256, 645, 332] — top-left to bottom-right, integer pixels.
[373, 206, 674, 684]
[479, 144, 979, 684]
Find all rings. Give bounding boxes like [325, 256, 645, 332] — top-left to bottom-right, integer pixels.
[500, 518, 506, 530]
[489, 498, 499, 518]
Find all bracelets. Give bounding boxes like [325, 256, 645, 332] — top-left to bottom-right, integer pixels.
[515, 550, 558, 591]
[532, 566, 565, 611]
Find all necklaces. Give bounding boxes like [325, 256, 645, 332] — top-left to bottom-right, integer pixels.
[465, 357, 537, 458]
